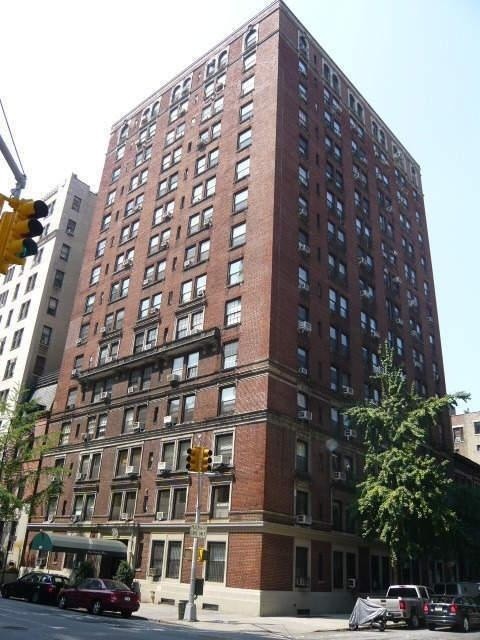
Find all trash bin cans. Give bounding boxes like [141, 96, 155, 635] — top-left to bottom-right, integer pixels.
[4, 572, 17, 584]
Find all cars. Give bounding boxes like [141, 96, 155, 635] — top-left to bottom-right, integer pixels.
[57, 579, 139, 617]
[1, 573, 70, 603]
[424, 595, 480, 631]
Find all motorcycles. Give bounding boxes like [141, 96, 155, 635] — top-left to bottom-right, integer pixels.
[348, 598, 386, 632]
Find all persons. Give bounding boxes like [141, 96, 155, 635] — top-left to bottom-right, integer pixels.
[6, 562, 19, 577]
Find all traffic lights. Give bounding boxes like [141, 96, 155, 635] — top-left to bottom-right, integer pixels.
[186, 447, 200, 472]
[200, 447, 212, 472]
[3, 198, 48, 266]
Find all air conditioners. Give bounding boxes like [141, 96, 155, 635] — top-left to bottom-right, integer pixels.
[299, 366, 310, 377]
[121, 513, 133, 520]
[36, 557, 47, 567]
[298, 242, 314, 256]
[97, 391, 110, 401]
[128, 420, 145, 431]
[160, 209, 173, 222]
[147, 568, 162, 577]
[77, 431, 91, 442]
[334, 467, 349, 487]
[301, 514, 313, 524]
[163, 373, 180, 382]
[96, 325, 108, 334]
[155, 460, 170, 471]
[390, 270, 427, 347]
[140, 278, 150, 286]
[299, 208, 310, 217]
[348, 577, 357, 589]
[359, 288, 371, 302]
[120, 258, 131, 269]
[157, 512, 168, 521]
[344, 427, 359, 441]
[147, 306, 159, 316]
[300, 320, 314, 335]
[352, 254, 368, 270]
[140, 343, 153, 351]
[159, 414, 178, 426]
[298, 279, 312, 295]
[102, 356, 115, 363]
[180, 259, 193, 268]
[210, 454, 225, 465]
[125, 385, 136, 394]
[123, 464, 141, 476]
[342, 384, 356, 394]
[195, 137, 206, 148]
[130, 204, 144, 213]
[158, 239, 169, 249]
[134, 137, 140, 146]
[73, 336, 84, 347]
[297, 409, 315, 423]
[195, 286, 206, 298]
[69, 368, 78, 378]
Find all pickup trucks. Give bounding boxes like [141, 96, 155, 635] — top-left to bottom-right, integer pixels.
[367, 584, 434, 628]
